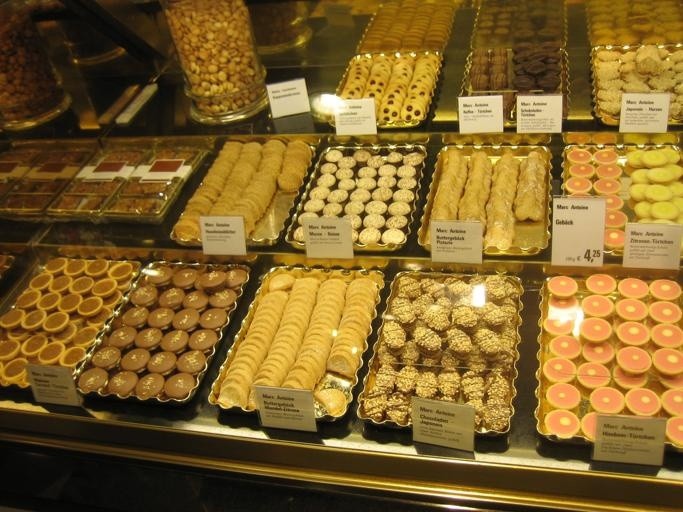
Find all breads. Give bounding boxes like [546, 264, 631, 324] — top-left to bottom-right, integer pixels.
[359, 272, 517, 433]
[1, 258, 136, 386]
[363, 2, 459, 50]
[0, 145, 94, 214]
[457, 148, 493, 223]
[484, 152, 515, 250]
[340, 54, 439, 122]
[217, 270, 378, 416]
[595, 41, 683, 120]
[54, 144, 148, 212]
[545, 276, 683, 449]
[176, 139, 310, 241]
[113, 143, 195, 213]
[467, 44, 566, 121]
[424, 149, 468, 244]
[624, 151, 683, 225]
[566, 147, 628, 248]
[479, 2, 564, 44]
[515, 151, 547, 221]
[590, 0, 683, 45]
[77, 266, 247, 397]
[297, 148, 422, 245]
